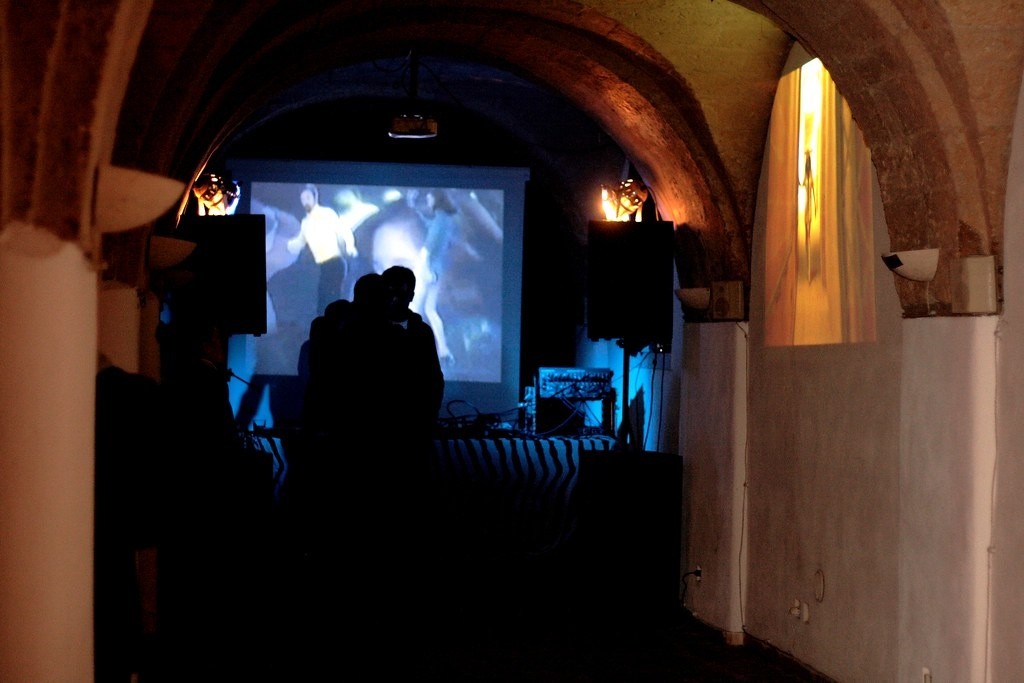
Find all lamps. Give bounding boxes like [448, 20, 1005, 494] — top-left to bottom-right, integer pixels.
[613, 177, 649, 219]
[192, 174, 226, 216]
[387, 47, 439, 140]
[881, 248, 939, 282]
[92, 162, 186, 238]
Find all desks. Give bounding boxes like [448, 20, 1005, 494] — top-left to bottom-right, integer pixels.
[434, 436, 619, 555]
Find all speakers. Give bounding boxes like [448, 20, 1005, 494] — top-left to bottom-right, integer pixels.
[585, 222, 674, 343]
[165, 214, 267, 337]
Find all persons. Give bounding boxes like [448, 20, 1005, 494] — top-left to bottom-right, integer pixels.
[249, 179, 503, 382]
[94, 263, 446, 658]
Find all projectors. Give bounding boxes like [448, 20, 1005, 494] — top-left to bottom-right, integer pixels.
[388, 117, 437, 138]
